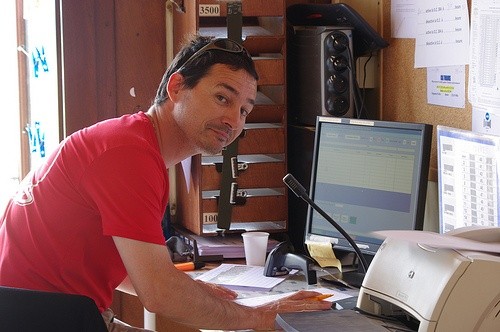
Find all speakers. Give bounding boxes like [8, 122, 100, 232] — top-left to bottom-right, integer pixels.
[287, 22, 356, 253]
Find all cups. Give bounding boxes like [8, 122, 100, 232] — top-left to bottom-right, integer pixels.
[242, 232, 269, 266]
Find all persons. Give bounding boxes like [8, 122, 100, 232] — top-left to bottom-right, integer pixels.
[0, 34, 334, 332]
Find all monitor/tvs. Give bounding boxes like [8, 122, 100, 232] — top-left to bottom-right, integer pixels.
[305, 116, 434, 289]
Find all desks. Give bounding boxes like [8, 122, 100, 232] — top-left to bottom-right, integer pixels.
[111, 259, 246, 332]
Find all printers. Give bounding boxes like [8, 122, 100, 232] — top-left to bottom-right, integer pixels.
[275, 225, 500, 332]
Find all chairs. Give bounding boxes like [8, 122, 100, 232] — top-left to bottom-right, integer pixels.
[0, 283, 108, 332]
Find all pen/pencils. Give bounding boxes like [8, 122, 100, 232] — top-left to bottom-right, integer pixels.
[303, 294, 334, 301]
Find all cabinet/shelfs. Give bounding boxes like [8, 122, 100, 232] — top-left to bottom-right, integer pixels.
[163, 0, 289, 236]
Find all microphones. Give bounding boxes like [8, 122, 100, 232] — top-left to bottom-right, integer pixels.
[282, 173, 369, 274]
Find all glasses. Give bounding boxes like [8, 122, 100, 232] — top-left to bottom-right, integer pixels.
[176, 38, 252, 72]
